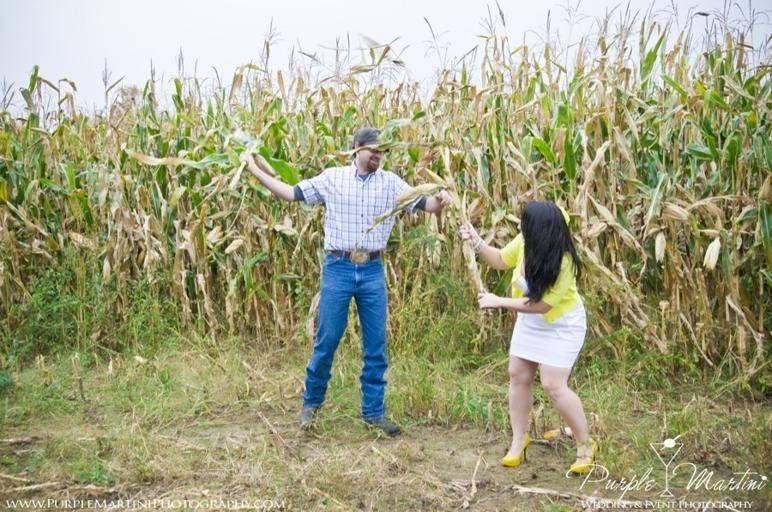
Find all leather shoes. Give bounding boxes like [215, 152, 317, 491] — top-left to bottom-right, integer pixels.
[364, 417, 401, 437]
[299, 406, 317, 430]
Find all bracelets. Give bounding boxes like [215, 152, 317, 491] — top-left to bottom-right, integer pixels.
[474, 239, 486, 254]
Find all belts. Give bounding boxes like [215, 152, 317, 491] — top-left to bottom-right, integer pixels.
[331, 250, 380, 264]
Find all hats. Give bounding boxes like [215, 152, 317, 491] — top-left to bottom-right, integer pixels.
[352, 128, 389, 152]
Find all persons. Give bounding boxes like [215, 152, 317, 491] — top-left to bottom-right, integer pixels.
[460, 199, 601, 476]
[236, 127, 455, 439]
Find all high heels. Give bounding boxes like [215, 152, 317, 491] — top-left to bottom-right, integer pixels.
[569, 439, 597, 474]
[500, 432, 530, 467]
